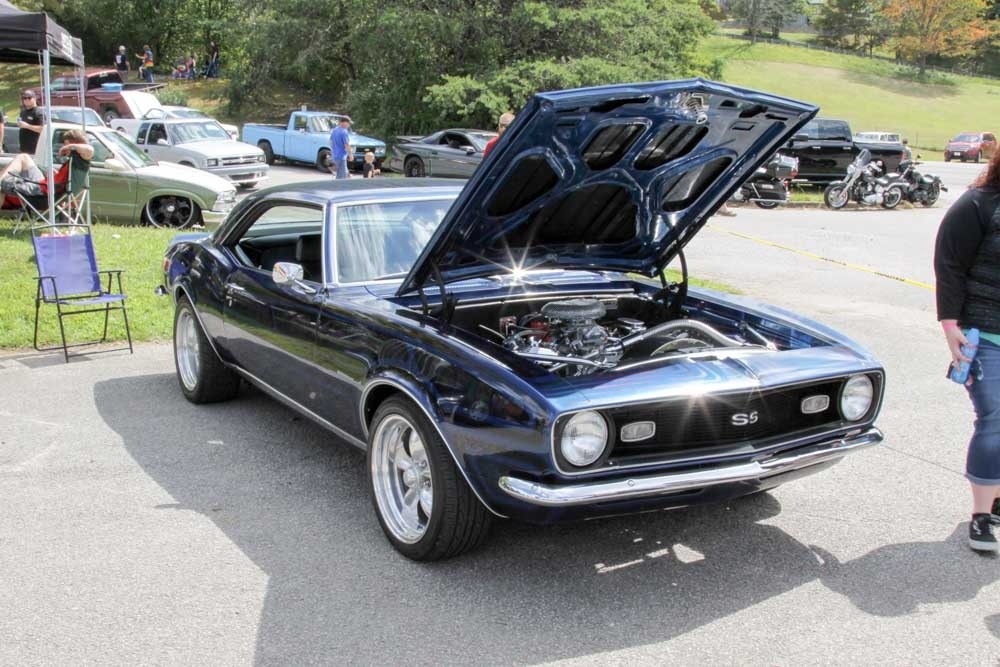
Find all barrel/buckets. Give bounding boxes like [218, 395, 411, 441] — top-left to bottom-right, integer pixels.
[101, 82, 123, 90]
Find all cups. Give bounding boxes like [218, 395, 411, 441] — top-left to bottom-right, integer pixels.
[18, 118, 22, 121]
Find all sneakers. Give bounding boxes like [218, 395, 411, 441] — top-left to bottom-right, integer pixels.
[991, 497, 1000, 524]
[968, 513, 1000, 551]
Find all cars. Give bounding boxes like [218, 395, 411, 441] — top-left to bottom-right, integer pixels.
[945, 133, 999, 164]
[389, 128, 498, 177]
[0, 68, 270, 231]
[155, 79, 884, 564]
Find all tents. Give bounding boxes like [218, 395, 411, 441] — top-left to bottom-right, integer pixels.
[0, 0, 93, 266]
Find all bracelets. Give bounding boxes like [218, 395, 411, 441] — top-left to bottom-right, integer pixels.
[943, 322, 956, 332]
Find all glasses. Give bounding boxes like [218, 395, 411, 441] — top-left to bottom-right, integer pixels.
[499, 124, 509, 129]
[23, 95, 31, 99]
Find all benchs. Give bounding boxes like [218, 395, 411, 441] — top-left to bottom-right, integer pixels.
[176, 71, 199, 80]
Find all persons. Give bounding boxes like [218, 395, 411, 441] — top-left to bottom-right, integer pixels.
[115, 45, 153, 83]
[170, 41, 218, 80]
[483, 112, 516, 159]
[0, 90, 94, 197]
[934, 141, 1000, 552]
[714, 202, 737, 217]
[364, 152, 380, 178]
[329, 115, 353, 179]
[901, 139, 912, 160]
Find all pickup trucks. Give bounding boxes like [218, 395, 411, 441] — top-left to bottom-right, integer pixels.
[780, 119, 913, 183]
[241, 111, 388, 173]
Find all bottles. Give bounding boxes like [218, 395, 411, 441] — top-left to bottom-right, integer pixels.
[949, 328, 980, 384]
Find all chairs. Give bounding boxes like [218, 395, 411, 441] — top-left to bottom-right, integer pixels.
[8, 152, 92, 236]
[294, 234, 336, 285]
[385, 227, 422, 273]
[31, 224, 133, 363]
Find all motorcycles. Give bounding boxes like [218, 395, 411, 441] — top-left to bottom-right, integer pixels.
[822, 149, 924, 211]
[741, 154, 799, 208]
[877, 159, 947, 210]
